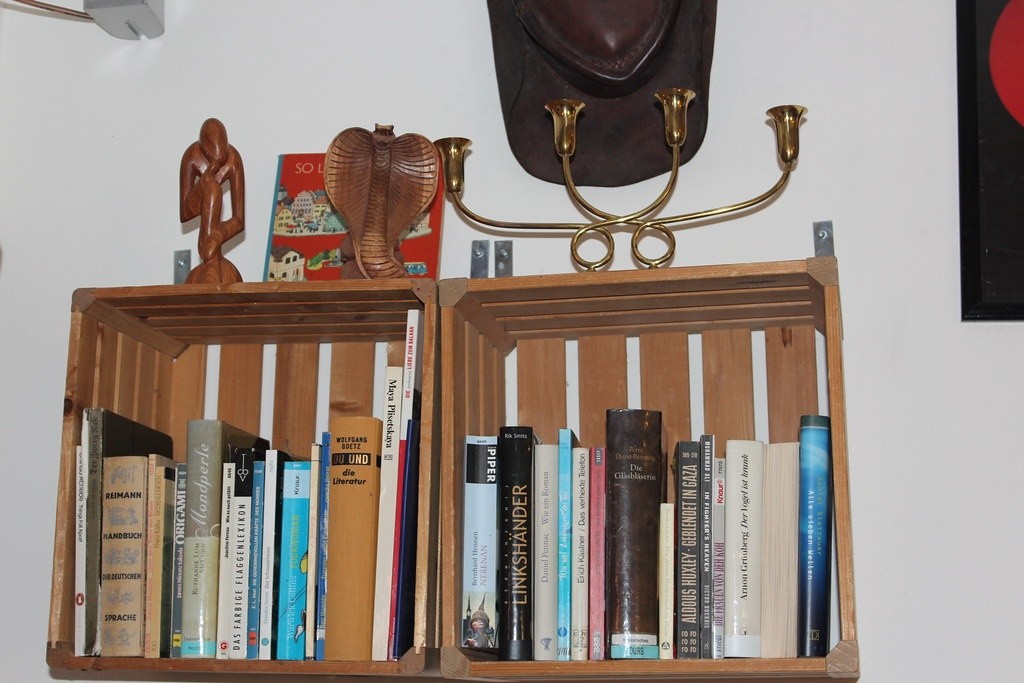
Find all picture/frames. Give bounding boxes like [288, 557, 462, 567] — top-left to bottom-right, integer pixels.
[956, 0, 1023, 321]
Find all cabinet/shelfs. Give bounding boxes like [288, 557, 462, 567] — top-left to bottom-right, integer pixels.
[45, 276, 440, 683]
[438, 256, 859, 681]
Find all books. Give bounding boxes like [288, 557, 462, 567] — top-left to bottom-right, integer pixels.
[672, 440, 701, 661]
[799, 414, 833, 657]
[500, 424, 541, 661]
[761, 441, 801, 661]
[459, 434, 499, 649]
[263, 153, 447, 280]
[558, 429, 583, 664]
[531, 443, 561, 662]
[606, 407, 667, 661]
[659, 502, 676, 661]
[569, 447, 588, 662]
[701, 433, 713, 661]
[589, 447, 604, 662]
[711, 457, 725, 661]
[725, 438, 765, 657]
[75, 310, 424, 662]
